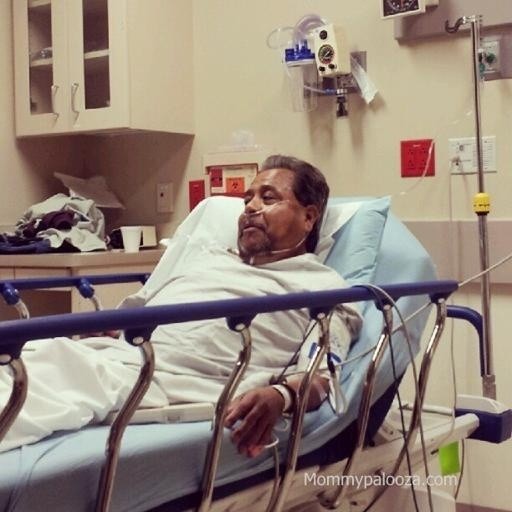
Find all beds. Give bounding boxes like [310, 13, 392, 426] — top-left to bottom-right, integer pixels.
[0, 196, 512, 511]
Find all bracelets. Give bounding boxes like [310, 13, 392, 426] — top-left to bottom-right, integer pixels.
[272, 379, 294, 417]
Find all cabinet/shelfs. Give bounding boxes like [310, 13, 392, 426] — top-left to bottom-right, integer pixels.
[10, 0, 196, 137]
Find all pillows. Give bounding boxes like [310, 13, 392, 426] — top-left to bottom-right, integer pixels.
[133, 197, 389, 304]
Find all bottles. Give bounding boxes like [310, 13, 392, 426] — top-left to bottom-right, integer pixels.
[284, 42, 318, 113]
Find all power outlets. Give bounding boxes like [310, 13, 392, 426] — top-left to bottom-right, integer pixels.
[224, 176, 244, 196]
[400, 140, 435, 178]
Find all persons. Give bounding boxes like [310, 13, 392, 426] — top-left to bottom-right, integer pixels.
[1, 153, 364, 459]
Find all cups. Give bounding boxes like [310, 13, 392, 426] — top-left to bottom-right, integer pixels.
[119, 225, 141, 255]
